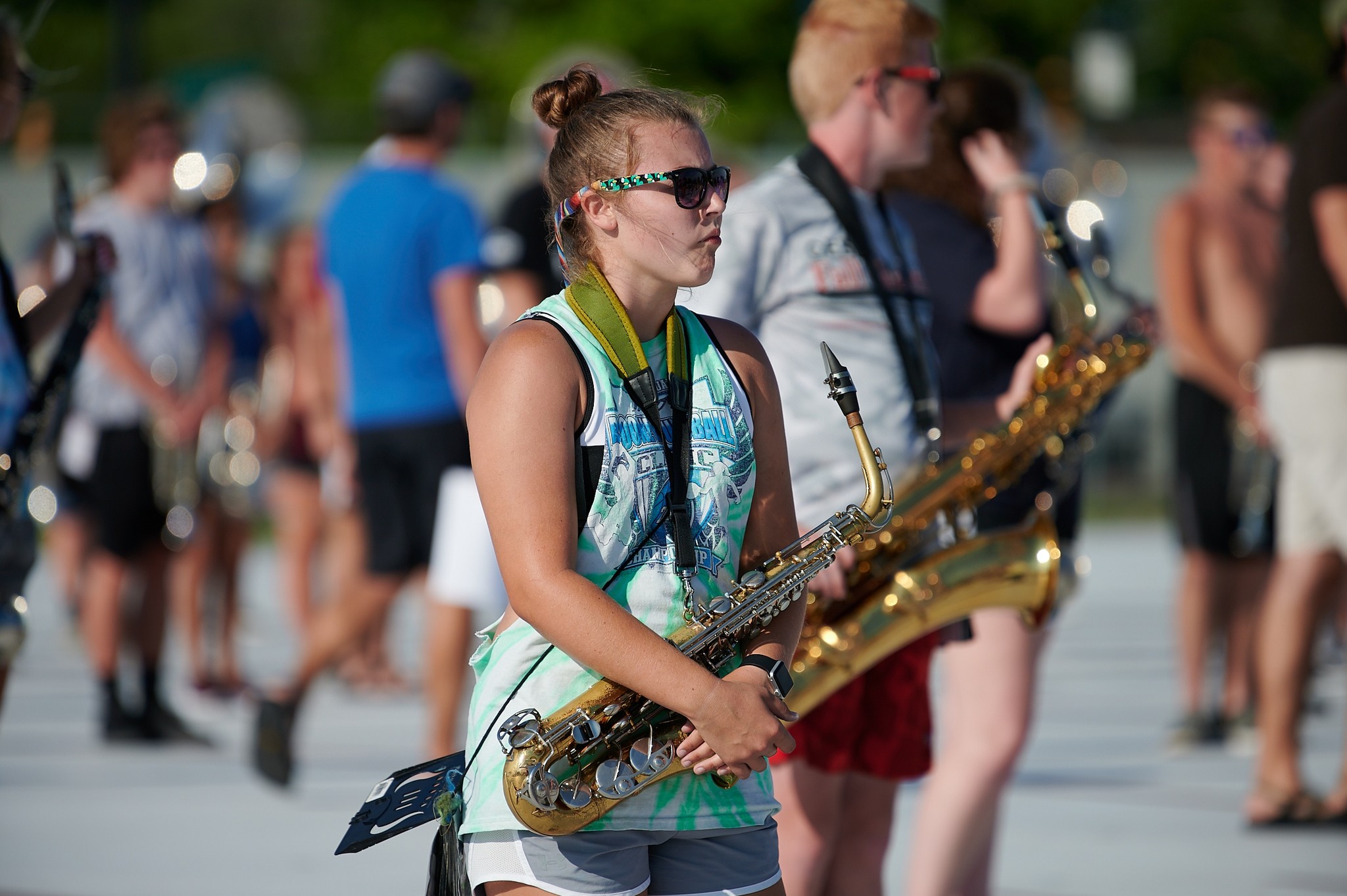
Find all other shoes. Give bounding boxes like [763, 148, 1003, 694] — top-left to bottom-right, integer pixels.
[97, 671, 176, 744]
[254, 699, 296, 787]
[134, 667, 195, 741]
[1180, 712, 1231, 749]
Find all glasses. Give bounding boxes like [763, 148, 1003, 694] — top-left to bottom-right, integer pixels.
[1199, 121, 1270, 154]
[852, 64, 942, 97]
[594, 166, 731, 209]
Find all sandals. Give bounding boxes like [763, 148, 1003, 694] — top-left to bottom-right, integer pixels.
[1251, 791, 1322, 825]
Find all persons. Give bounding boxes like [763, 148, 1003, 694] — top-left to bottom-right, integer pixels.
[1152, 15, 1347, 832]
[464, 67, 808, 896]
[677, 0, 1096, 896]
[0, 41, 639, 785]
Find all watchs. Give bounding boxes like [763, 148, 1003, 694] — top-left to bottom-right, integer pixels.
[737, 654, 794, 701]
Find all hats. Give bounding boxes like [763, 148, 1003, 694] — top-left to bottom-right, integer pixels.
[374, 52, 472, 116]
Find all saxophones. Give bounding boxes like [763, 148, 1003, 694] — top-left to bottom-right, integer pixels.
[496, 342, 895, 839]
[771, 192, 1167, 733]
